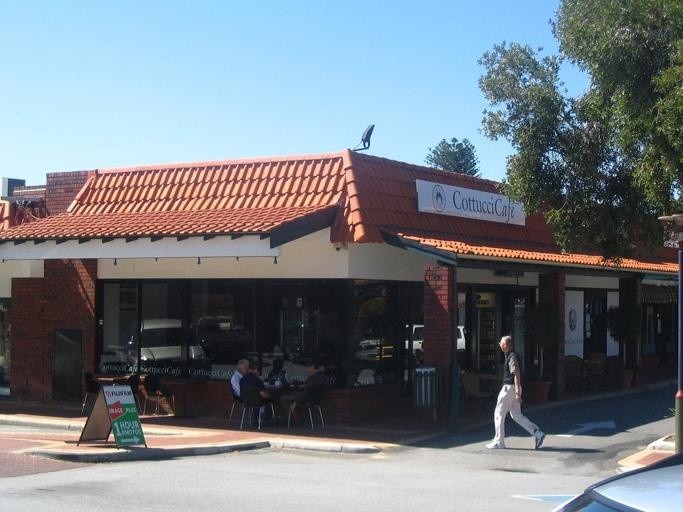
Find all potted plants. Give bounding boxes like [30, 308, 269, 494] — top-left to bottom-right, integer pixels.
[604, 304, 642, 388]
[525, 309, 561, 402]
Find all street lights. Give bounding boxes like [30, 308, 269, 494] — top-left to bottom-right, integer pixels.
[657, 213, 683, 454]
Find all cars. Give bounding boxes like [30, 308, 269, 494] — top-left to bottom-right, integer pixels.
[125, 318, 212, 379]
[197, 316, 252, 356]
[354, 340, 397, 363]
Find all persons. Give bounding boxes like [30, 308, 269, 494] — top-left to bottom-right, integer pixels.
[291, 359, 326, 426]
[239, 362, 276, 426]
[268, 357, 288, 386]
[233, 359, 249, 396]
[486, 335, 545, 449]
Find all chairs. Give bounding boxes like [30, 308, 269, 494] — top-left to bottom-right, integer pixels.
[229, 368, 328, 430]
[82, 370, 175, 416]
[461, 352, 620, 419]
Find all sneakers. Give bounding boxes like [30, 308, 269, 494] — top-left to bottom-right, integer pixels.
[485, 442, 505, 448]
[535, 430, 545, 449]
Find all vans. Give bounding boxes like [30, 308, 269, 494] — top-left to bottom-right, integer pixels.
[404, 323, 466, 366]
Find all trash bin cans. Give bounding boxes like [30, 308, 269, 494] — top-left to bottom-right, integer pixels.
[413, 366, 438, 425]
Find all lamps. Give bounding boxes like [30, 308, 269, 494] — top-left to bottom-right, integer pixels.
[353, 123, 375, 151]
